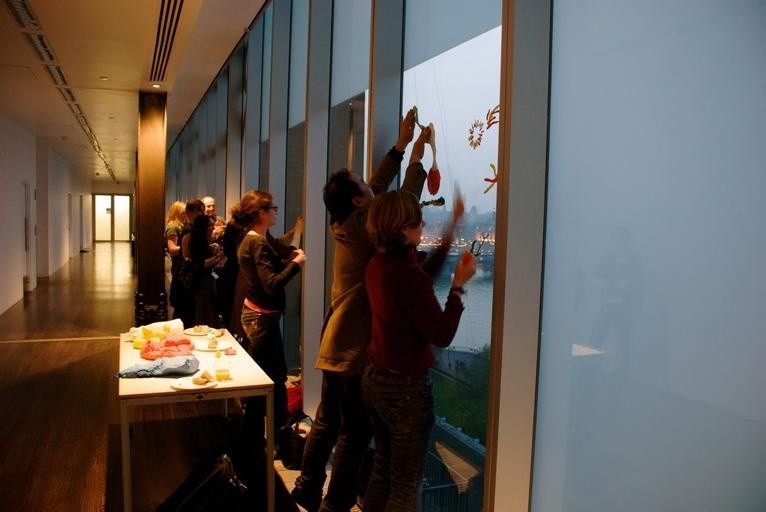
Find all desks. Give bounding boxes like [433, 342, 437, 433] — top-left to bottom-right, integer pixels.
[118, 326, 276, 512]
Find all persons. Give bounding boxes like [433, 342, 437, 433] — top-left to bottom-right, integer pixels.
[289, 107, 476, 511]
[164, 189, 307, 470]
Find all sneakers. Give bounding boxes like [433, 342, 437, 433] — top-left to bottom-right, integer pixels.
[290, 486, 322, 511]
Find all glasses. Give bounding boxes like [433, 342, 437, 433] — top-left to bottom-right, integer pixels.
[266, 205, 279, 212]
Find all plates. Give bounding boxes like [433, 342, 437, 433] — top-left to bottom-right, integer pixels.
[183, 325, 216, 336]
[170, 375, 219, 391]
[191, 338, 232, 352]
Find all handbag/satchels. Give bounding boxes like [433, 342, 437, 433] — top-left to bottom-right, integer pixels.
[157, 452, 250, 512]
[279, 414, 315, 469]
[176, 264, 200, 287]
